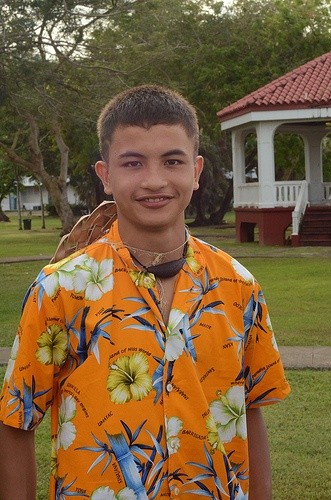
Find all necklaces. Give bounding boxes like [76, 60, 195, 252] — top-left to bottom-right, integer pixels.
[128, 231, 188, 278]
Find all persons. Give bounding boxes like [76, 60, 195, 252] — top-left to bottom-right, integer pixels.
[1, 85, 290, 500]
